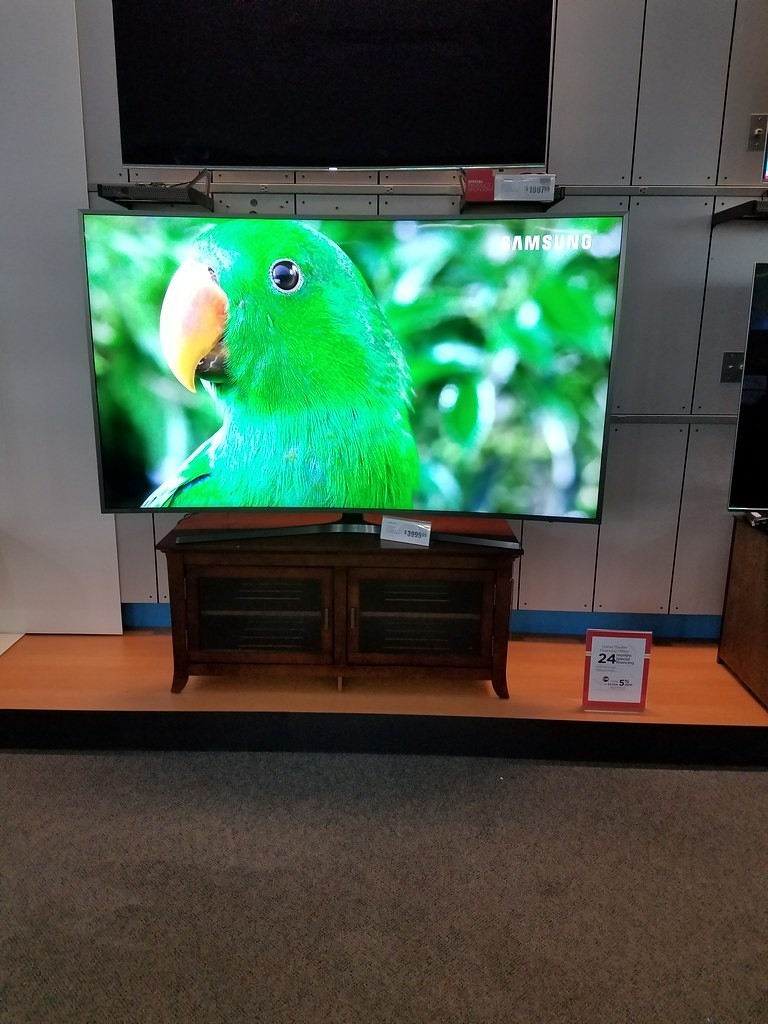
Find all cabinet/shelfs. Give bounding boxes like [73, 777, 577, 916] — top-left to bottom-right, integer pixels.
[155, 513, 524, 699]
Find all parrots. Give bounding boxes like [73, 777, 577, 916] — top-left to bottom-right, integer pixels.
[138, 220, 419, 509]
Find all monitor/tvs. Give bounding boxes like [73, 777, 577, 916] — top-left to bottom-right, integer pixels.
[727, 261, 768, 513]
[74, 206, 632, 554]
[111, 0, 558, 173]
